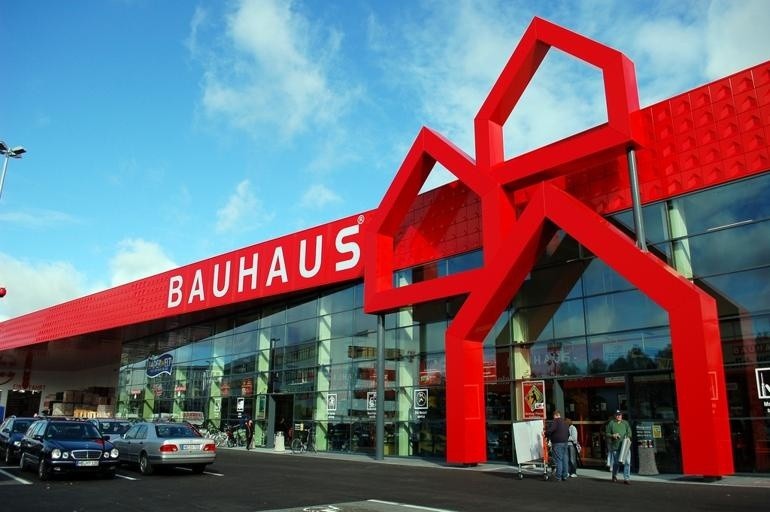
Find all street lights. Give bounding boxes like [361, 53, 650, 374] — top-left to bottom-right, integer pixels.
[0, 141, 27, 199]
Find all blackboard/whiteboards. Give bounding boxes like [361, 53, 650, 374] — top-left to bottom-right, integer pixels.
[512, 419, 545, 464]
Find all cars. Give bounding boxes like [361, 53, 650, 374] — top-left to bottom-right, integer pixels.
[0, 416, 216, 480]
[288, 418, 392, 447]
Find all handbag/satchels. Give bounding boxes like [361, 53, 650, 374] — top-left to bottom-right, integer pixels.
[573, 443, 582, 456]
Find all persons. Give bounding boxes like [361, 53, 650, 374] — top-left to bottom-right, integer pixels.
[244, 418, 254, 450]
[605, 408, 634, 486]
[565, 416, 581, 479]
[541, 410, 570, 482]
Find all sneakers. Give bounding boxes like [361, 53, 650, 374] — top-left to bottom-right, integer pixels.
[556, 473, 578, 481]
[623, 480, 634, 485]
[612, 477, 617, 483]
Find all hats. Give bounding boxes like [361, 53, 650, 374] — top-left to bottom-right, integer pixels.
[614, 409, 623, 415]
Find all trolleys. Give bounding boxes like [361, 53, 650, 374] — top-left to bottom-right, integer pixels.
[518, 438, 552, 481]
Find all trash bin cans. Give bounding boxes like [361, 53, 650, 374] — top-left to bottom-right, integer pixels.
[637, 440, 659, 475]
[274, 431, 285, 452]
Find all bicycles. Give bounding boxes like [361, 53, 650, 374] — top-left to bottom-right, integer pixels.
[205, 426, 255, 447]
[291, 430, 317, 454]
